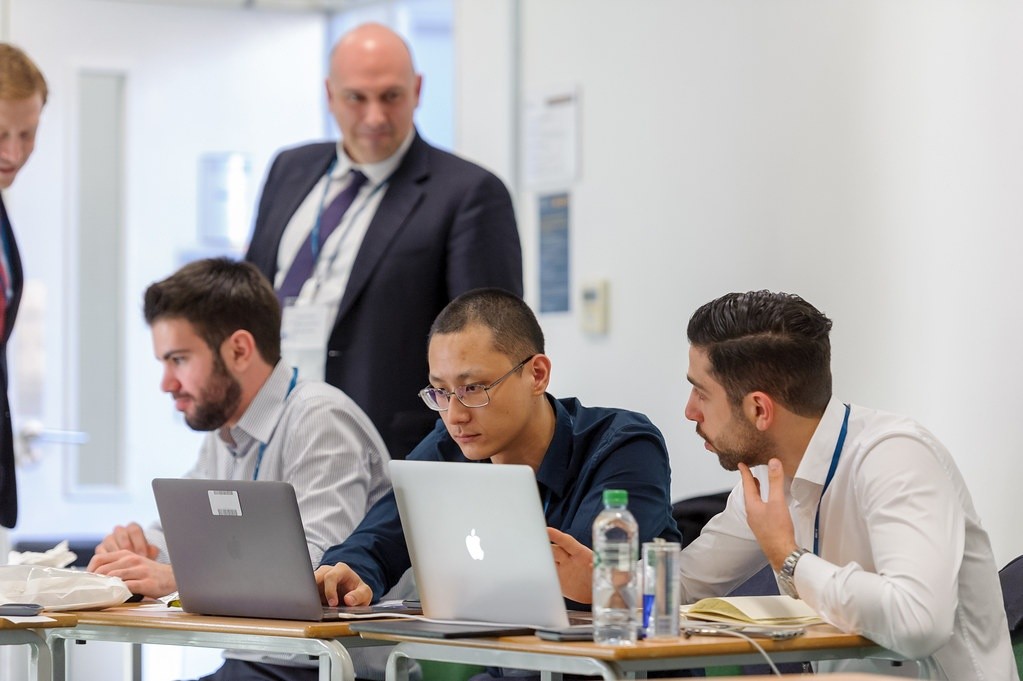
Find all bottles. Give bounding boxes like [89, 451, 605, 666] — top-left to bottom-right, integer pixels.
[592, 490, 639, 646]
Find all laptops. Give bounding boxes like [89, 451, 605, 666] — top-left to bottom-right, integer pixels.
[389, 460, 713, 634]
[152, 478, 424, 621]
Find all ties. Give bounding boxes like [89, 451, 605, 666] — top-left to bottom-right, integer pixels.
[0, 262, 7, 340]
[276, 170, 368, 306]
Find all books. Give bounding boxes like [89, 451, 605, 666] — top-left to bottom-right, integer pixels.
[677, 595, 827, 628]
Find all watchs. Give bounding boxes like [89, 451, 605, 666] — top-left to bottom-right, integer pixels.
[775, 548, 812, 600]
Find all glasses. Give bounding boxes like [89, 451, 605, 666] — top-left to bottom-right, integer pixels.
[416, 355, 537, 411]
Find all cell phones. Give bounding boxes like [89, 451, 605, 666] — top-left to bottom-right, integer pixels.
[535, 626, 647, 642]
[685, 622, 804, 640]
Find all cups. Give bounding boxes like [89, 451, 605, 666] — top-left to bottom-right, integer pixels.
[641, 542, 682, 638]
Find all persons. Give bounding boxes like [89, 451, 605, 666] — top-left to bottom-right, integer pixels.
[545, 291, 1019, 681]
[309, 289, 684, 681]
[0, 43, 50, 533]
[82, 257, 419, 681]
[246, 22, 523, 460]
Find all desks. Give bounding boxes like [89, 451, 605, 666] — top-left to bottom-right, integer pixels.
[359, 609, 947, 681]
[0, 602, 428, 681]
